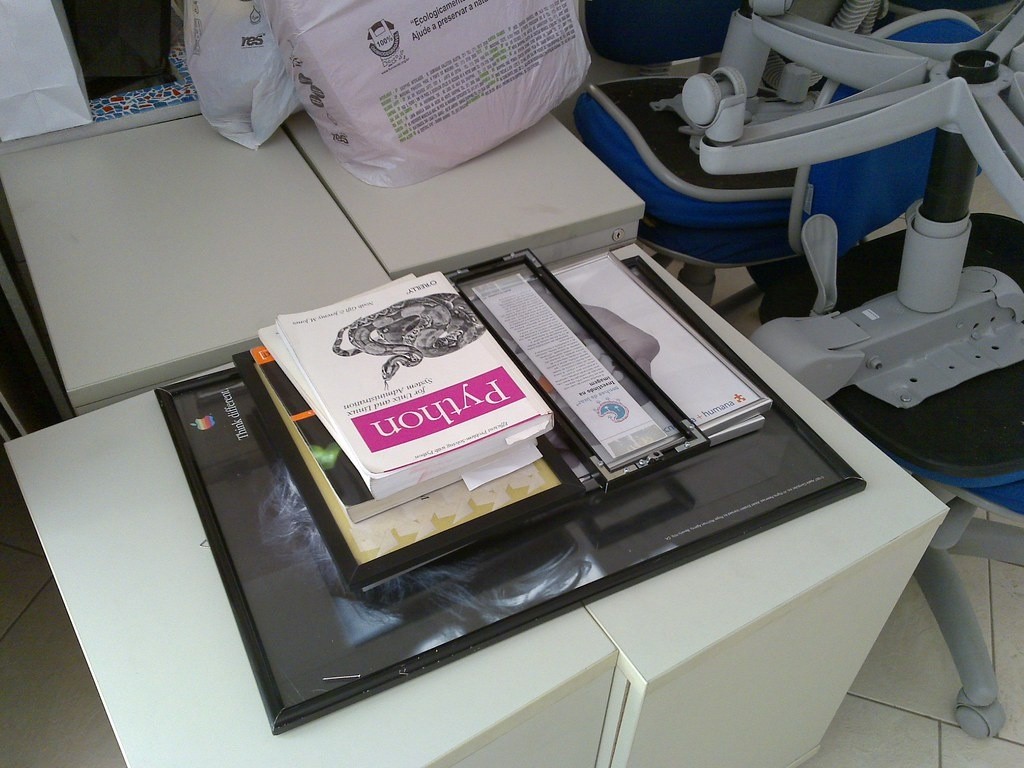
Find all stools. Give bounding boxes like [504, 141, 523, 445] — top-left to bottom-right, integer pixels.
[574, 0, 1024, 739]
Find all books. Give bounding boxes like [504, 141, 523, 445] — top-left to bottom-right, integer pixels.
[230, 247, 774, 592]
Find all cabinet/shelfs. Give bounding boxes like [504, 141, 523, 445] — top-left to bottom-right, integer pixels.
[0, 364, 619, 768]
[0, 113, 392, 410]
[285, 112, 644, 277]
[553, 245, 947, 768]
